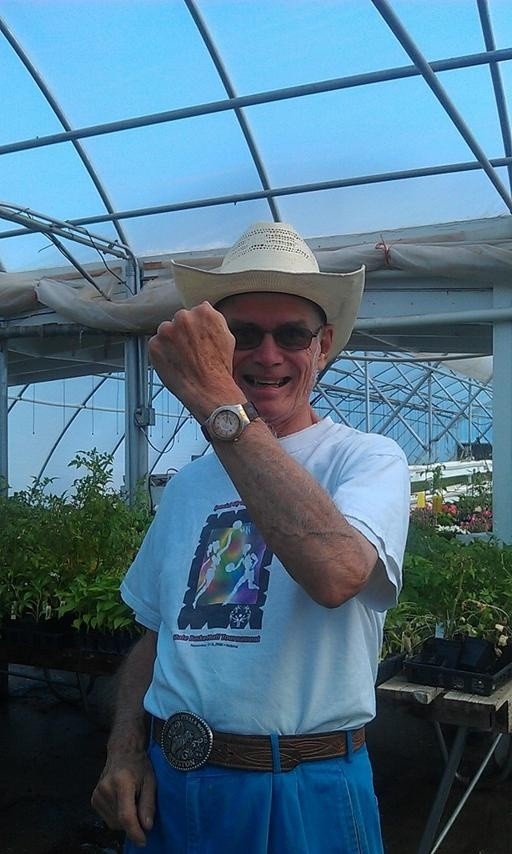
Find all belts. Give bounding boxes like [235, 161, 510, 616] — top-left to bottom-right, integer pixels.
[150, 712, 365, 774]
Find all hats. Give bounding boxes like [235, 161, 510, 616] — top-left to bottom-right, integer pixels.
[172, 222, 366, 363]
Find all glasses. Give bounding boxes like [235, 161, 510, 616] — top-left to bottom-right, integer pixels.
[232, 322, 325, 352]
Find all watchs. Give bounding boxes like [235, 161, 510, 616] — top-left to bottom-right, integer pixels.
[201, 401, 260, 444]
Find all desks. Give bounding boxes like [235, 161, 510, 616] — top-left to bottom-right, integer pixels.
[376, 668, 512, 854]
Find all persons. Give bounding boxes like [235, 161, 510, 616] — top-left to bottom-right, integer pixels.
[88, 223, 413, 854]
